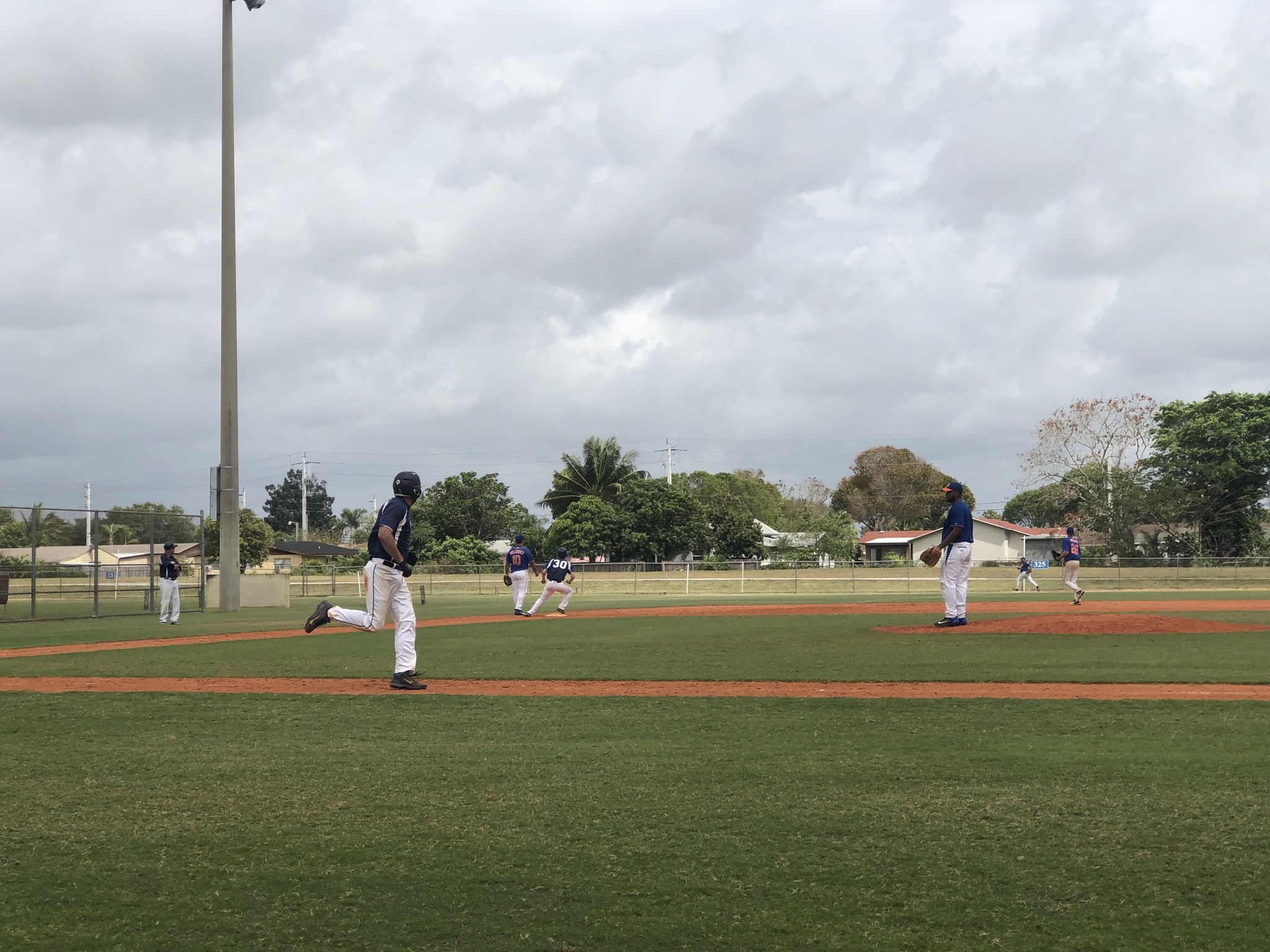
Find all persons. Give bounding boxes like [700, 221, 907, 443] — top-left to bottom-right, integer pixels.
[1013, 557, 1040, 592]
[1056, 527, 1084, 606]
[505, 534, 539, 616]
[304, 471, 427, 690]
[159, 541, 182, 626]
[524, 547, 575, 617]
[920, 482, 973, 628]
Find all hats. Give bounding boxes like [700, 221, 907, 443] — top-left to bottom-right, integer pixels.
[941, 483, 963, 492]
[1020, 557, 1025, 560]
[515, 535, 524, 542]
[1067, 527, 1073, 532]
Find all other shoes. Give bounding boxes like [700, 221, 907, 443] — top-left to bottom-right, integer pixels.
[524, 613, 531, 617]
[557, 608, 564, 614]
[515, 609, 525, 616]
[1012, 588, 1018, 591]
[1038, 585, 1040, 592]
[1070, 600, 1082, 605]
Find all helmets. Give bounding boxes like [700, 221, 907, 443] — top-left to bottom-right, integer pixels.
[558, 547, 567, 558]
[393, 471, 424, 497]
[164, 542, 178, 549]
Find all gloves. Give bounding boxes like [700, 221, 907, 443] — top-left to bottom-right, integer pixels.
[406, 552, 418, 567]
[399, 559, 412, 577]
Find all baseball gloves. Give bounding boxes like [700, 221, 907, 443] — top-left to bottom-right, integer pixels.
[1051, 549, 1062, 562]
[503, 575, 512, 587]
[1026, 569, 1032, 574]
[918, 544, 942, 568]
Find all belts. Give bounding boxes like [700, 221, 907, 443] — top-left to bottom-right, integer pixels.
[164, 577, 176, 581]
[549, 578, 561, 583]
[369, 557, 399, 570]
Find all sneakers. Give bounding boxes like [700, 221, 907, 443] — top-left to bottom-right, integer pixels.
[1076, 590, 1084, 598]
[934, 617, 959, 626]
[390, 670, 427, 689]
[161, 622, 166, 625]
[959, 618, 967, 626]
[171, 621, 181, 625]
[305, 600, 333, 633]
